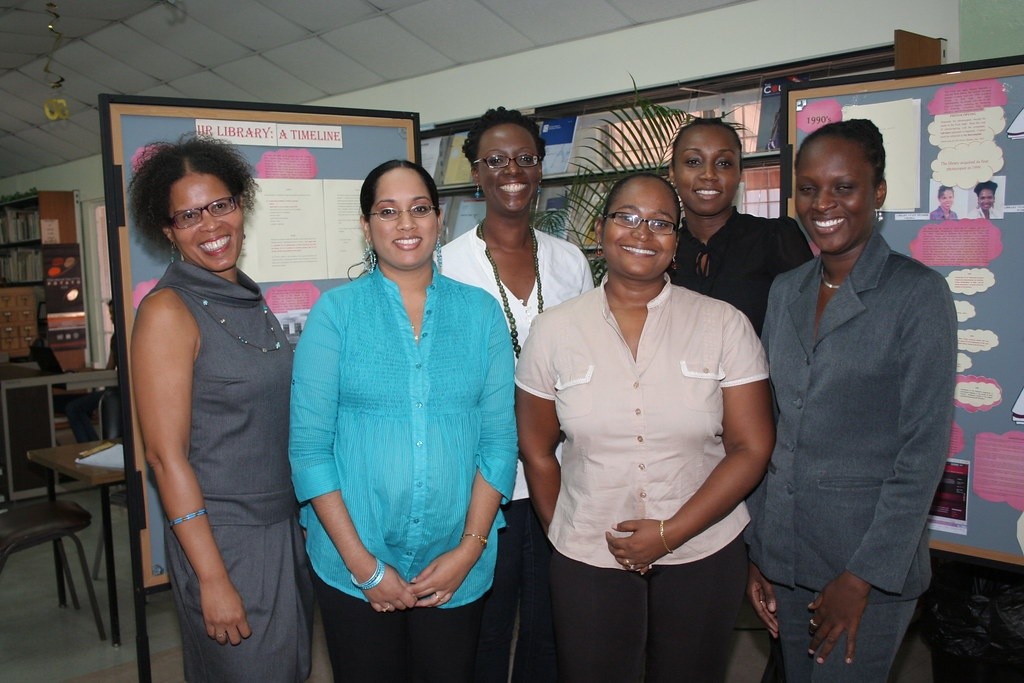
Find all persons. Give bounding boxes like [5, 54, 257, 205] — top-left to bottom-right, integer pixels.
[286, 159, 518, 683]
[930, 184, 959, 220]
[668, 117, 815, 338]
[431, 107, 597, 682]
[748, 119, 957, 683]
[969, 181, 998, 219]
[126, 133, 317, 683]
[65, 299, 116, 443]
[514, 173, 775, 683]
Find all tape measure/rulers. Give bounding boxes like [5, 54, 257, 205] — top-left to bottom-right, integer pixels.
[79, 441, 113, 459]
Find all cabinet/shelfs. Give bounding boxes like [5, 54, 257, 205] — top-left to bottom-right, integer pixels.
[0, 190, 88, 374]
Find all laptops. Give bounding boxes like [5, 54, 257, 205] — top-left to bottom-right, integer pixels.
[28, 346, 94, 374]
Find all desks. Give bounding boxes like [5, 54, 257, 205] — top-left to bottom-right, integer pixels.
[26, 439, 126, 647]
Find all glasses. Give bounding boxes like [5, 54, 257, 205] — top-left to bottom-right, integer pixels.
[602, 211, 677, 236]
[166, 194, 236, 229]
[470, 154, 543, 170]
[368, 203, 436, 221]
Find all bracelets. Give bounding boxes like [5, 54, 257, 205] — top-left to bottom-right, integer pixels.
[169, 509, 207, 526]
[349, 558, 386, 590]
[462, 534, 487, 548]
[659, 519, 674, 553]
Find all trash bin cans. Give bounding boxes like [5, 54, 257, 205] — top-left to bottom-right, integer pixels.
[922, 561, 1024, 683]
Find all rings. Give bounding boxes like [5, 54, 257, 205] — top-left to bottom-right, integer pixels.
[625, 558, 631, 566]
[759, 600, 766, 603]
[434, 594, 441, 601]
[809, 618, 819, 627]
[214, 631, 226, 638]
[381, 603, 390, 612]
[628, 565, 634, 570]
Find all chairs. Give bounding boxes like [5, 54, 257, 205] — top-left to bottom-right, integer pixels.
[0, 500, 107, 642]
[93, 388, 125, 580]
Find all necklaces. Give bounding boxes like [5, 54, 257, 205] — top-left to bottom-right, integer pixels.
[820, 265, 840, 288]
[187, 295, 283, 353]
[479, 217, 544, 357]
